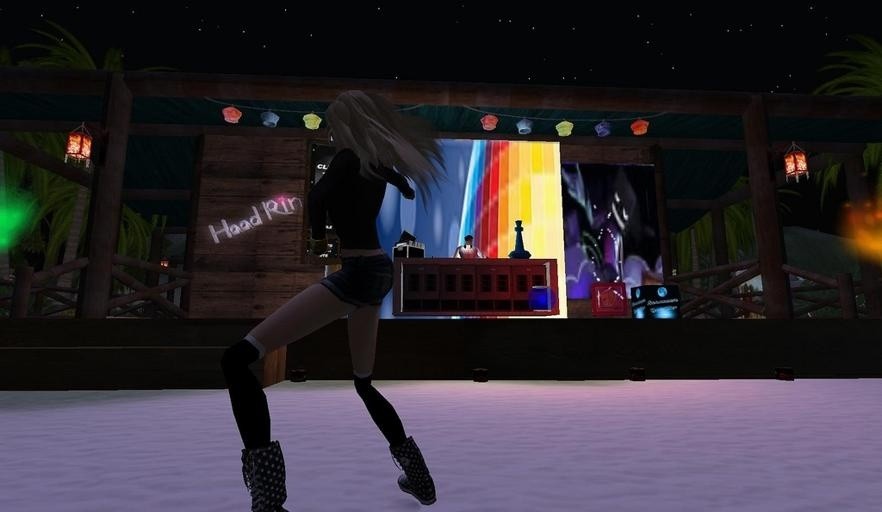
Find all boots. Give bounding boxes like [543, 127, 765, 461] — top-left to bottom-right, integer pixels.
[239, 439, 290, 512]
[387, 435, 438, 507]
